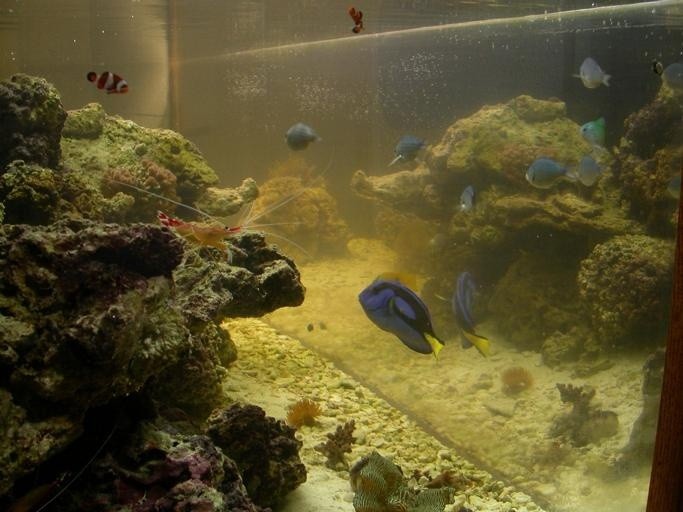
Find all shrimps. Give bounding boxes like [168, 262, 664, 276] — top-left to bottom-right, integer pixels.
[110, 152, 338, 267]
